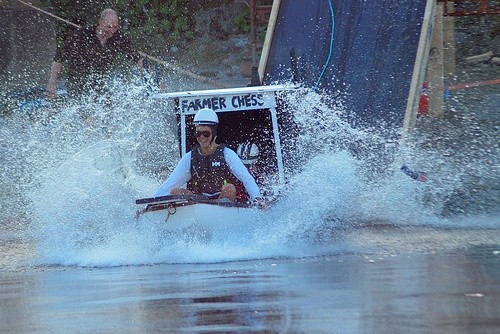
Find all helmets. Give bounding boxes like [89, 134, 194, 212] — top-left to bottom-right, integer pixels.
[191, 108, 219, 126]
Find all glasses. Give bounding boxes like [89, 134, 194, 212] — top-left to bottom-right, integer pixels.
[194, 131, 210, 138]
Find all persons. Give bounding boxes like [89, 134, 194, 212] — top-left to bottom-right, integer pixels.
[150, 107, 276, 211]
[45, 9, 154, 135]
